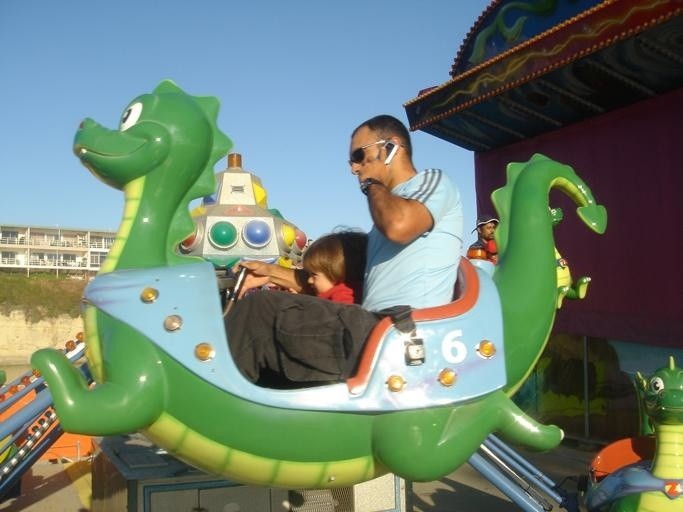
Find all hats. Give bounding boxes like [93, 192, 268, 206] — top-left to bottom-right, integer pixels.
[476, 215, 499, 228]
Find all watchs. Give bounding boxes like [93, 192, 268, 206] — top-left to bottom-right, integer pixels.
[360, 178, 385, 196]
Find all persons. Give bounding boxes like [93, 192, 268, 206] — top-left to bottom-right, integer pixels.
[302, 232, 365, 304]
[483, 240, 498, 264]
[223, 115, 464, 382]
[467, 213, 500, 249]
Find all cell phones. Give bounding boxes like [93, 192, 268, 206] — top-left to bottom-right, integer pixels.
[377, 141, 399, 165]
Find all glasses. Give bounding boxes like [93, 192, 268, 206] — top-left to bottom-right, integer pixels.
[348, 140, 387, 164]
